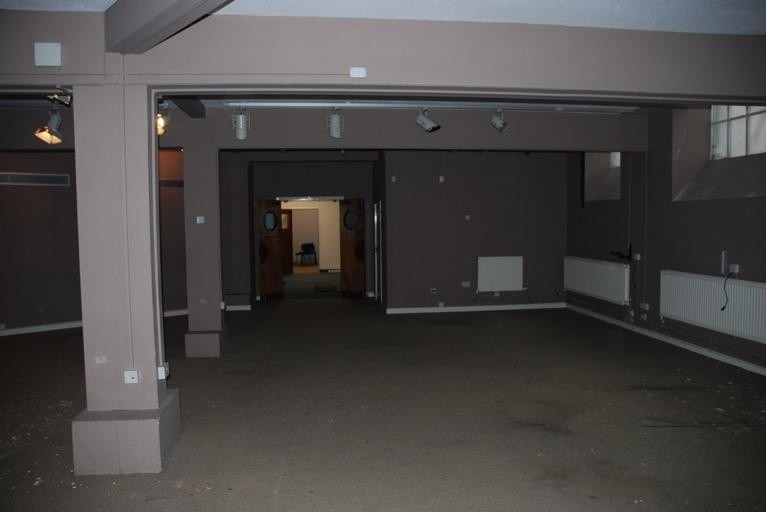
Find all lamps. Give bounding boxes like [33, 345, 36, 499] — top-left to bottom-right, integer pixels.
[33, 93, 174, 147]
[201, 93, 641, 143]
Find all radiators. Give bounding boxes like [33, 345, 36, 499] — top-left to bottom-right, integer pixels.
[476, 256, 525, 293]
[658, 268, 766, 346]
[562, 255, 633, 309]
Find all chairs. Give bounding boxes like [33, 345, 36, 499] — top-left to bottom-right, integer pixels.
[294, 242, 318, 268]
